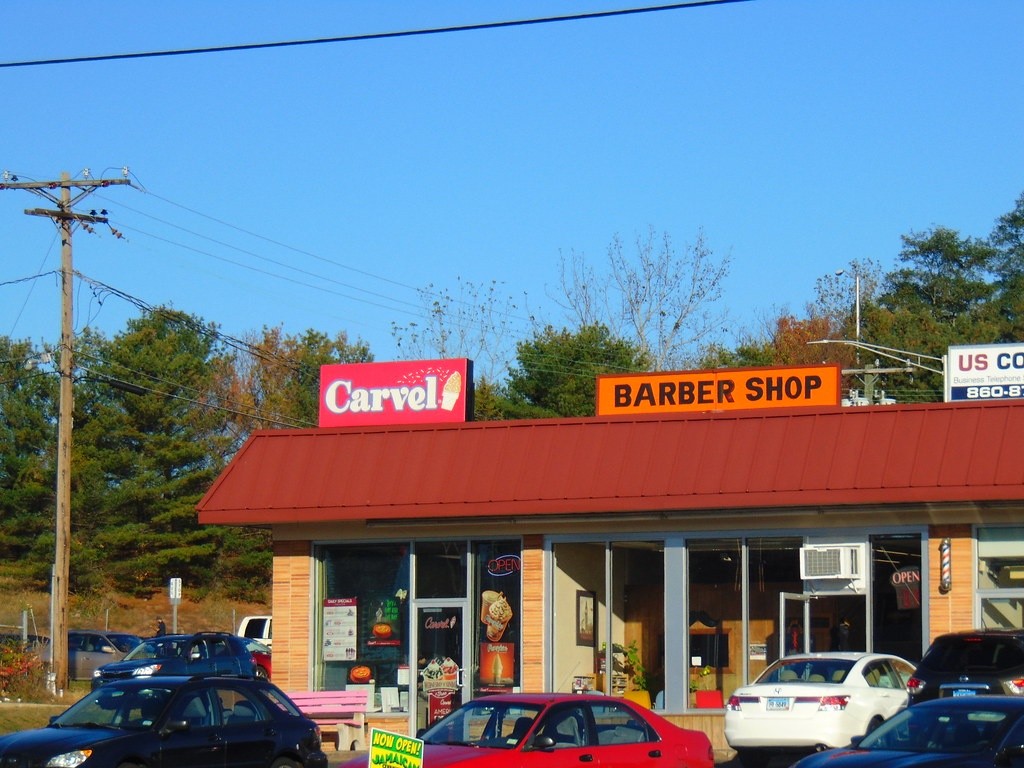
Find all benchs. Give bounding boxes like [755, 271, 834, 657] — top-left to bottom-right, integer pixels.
[274, 690, 369, 751]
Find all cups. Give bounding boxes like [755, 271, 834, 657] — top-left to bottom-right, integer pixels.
[487, 602, 512, 641]
[481, 591, 502, 624]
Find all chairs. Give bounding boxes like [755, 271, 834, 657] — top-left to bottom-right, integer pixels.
[232, 700, 255, 722]
[180, 697, 205, 725]
[779, 666, 879, 684]
[513, 717, 535, 746]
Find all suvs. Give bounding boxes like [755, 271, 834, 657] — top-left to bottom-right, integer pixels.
[905, 624, 1024, 741]
[89, 629, 259, 710]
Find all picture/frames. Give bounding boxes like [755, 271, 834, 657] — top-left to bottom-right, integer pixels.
[576, 590, 596, 647]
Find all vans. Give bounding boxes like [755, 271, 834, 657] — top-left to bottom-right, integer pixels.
[237, 614, 272, 653]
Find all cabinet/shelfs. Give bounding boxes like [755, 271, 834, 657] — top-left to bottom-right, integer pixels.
[591, 673, 628, 697]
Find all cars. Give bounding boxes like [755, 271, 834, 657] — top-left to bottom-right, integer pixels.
[0, 632, 52, 666]
[0, 676, 329, 768]
[237, 634, 272, 683]
[790, 691, 1024, 768]
[342, 691, 717, 768]
[68, 628, 157, 680]
[720, 652, 918, 768]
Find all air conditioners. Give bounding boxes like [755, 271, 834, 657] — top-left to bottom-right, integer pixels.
[798, 547, 859, 580]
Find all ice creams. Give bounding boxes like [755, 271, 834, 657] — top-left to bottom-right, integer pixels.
[481, 591, 512, 642]
[493, 654, 503, 684]
[422, 656, 458, 680]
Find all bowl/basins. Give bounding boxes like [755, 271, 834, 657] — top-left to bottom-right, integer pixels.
[350, 666, 371, 683]
[373, 624, 392, 639]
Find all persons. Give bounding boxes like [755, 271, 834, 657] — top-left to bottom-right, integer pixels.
[827, 617, 865, 652]
[148, 616, 166, 638]
[785, 619, 804, 656]
